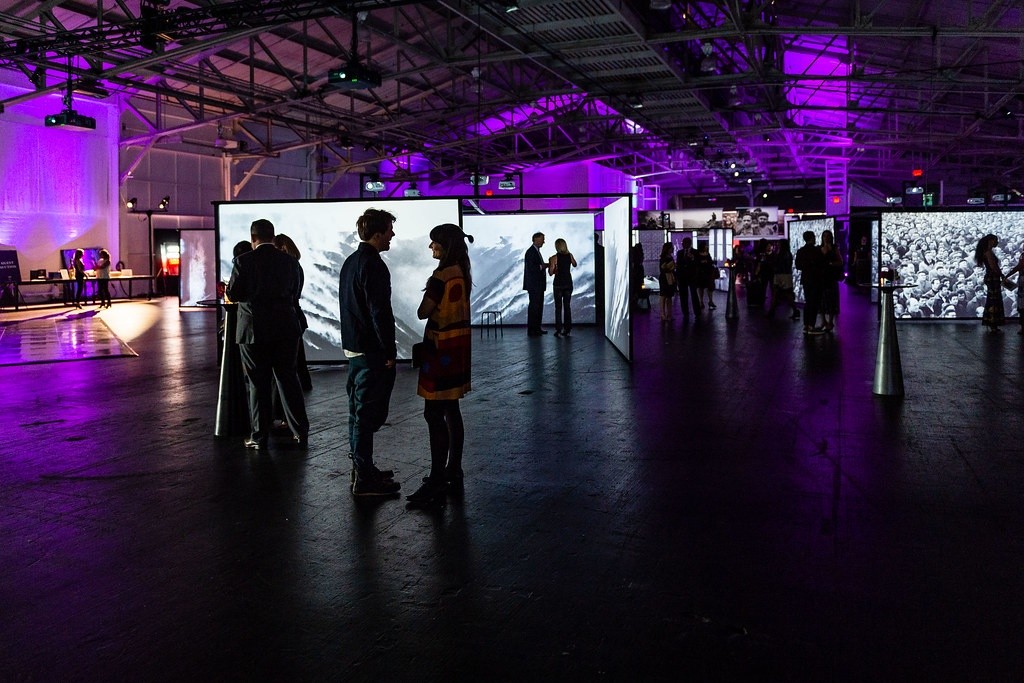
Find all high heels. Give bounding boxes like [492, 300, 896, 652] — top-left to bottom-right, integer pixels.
[708, 302, 717, 307]
[701, 303, 705, 309]
[666, 314, 675, 321]
[818, 321, 828, 331]
[661, 314, 665, 321]
[824, 325, 835, 334]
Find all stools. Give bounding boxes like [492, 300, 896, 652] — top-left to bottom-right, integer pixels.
[481, 311, 503, 339]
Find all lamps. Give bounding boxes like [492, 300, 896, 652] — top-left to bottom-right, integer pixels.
[728, 164, 768, 198]
[328, 69, 347, 80]
[503, 0, 520, 13]
[158, 196, 171, 211]
[1000, 107, 1011, 118]
[764, 134, 770, 142]
[126, 198, 137, 211]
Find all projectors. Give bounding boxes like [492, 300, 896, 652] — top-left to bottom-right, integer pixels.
[365, 182, 386, 192]
[471, 175, 489, 185]
[992, 190, 1011, 201]
[902, 180, 924, 194]
[885, 194, 903, 204]
[327, 68, 383, 91]
[404, 190, 420, 197]
[499, 181, 516, 190]
[966, 188, 985, 205]
[45, 114, 96, 131]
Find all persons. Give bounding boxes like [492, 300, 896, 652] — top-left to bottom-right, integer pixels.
[789, 218, 835, 303]
[92, 249, 111, 309]
[522, 232, 549, 337]
[548, 239, 577, 337]
[852, 235, 870, 284]
[676, 237, 702, 319]
[735, 208, 774, 236]
[1005, 248, 1024, 335]
[975, 234, 1005, 332]
[594, 233, 604, 302]
[697, 241, 717, 309]
[227, 219, 311, 450]
[816, 229, 842, 332]
[73, 249, 88, 309]
[735, 239, 800, 321]
[405, 223, 474, 503]
[339, 209, 401, 496]
[659, 242, 677, 321]
[871, 211, 1024, 319]
[795, 230, 829, 335]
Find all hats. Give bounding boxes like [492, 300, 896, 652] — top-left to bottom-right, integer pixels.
[430, 224, 474, 244]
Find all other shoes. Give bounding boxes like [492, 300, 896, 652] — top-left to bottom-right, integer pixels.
[808, 328, 825, 335]
[541, 329, 548, 334]
[353, 478, 401, 495]
[373, 466, 394, 476]
[554, 329, 562, 337]
[244, 435, 260, 450]
[528, 332, 542, 336]
[406, 477, 447, 500]
[562, 328, 571, 335]
[444, 477, 464, 497]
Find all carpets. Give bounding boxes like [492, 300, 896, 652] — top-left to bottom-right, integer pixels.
[0, 316, 139, 364]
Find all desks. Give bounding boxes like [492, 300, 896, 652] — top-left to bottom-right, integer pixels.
[19, 274, 155, 300]
[859, 283, 919, 396]
[198, 300, 239, 437]
